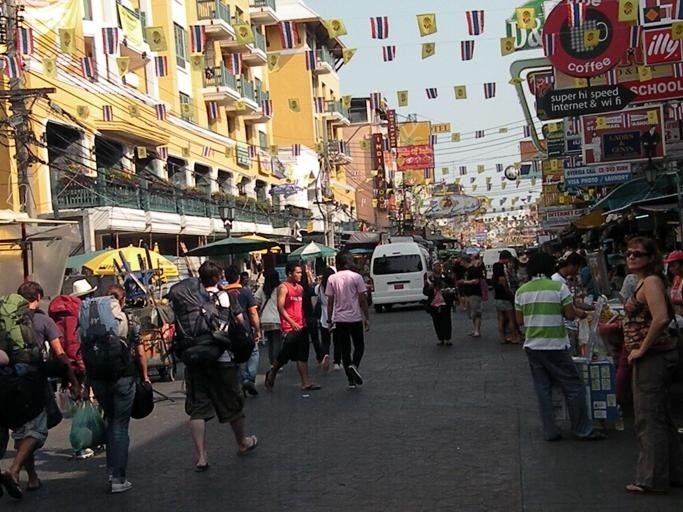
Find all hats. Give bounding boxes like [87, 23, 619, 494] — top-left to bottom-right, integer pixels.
[68, 278, 98, 297]
[662, 249, 683, 264]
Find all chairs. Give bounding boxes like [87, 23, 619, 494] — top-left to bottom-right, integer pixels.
[61, 270, 155, 308]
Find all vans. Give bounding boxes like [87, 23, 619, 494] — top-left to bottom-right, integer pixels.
[481, 247, 517, 289]
[368, 241, 432, 312]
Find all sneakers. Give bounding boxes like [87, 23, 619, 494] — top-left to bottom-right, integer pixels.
[110, 478, 133, 494]
[315, 353, 343, 371]
[244, 382, 259, 397]
[346, 364, 364, 390]
[577, 430, 610, 442]
[435, 340, 455, 346]
[468, 328, 482, 339]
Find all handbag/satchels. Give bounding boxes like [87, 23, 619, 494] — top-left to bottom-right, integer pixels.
[130, 382, 155, 420]
[228, 317, 257, 366]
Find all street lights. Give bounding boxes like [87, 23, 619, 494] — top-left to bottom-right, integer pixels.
[217, 198, 234, 268]
[321, 119, 387, 269]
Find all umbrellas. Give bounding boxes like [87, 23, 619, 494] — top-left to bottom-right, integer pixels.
[240, 233, 282, 255]
[184, 235, 270, 266]
[287, 241, 335, 274]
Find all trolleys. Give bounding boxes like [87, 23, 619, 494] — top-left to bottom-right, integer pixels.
[125, 303, 184, 382]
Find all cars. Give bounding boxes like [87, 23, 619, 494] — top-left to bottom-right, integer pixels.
[463, 246, 479, 255]
[511, 244, 525, 258]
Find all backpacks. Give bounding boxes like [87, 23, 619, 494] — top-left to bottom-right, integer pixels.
[65, 295, 129, 382]
[167, 276, 227, 365]
[0, 292, 44, 364]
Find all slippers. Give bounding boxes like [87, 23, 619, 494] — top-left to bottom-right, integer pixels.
[25, 479, 42, 493]
[195, 463, 208, 473]
[236, 434, 260, 458]
[0, 470, 24, 501]
[265, 370, 276, 393]
[300, 382, 322, 392]
[624, 482, 669, 497]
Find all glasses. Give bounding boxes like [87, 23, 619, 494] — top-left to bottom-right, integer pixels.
[624, 250, 649, 258]
